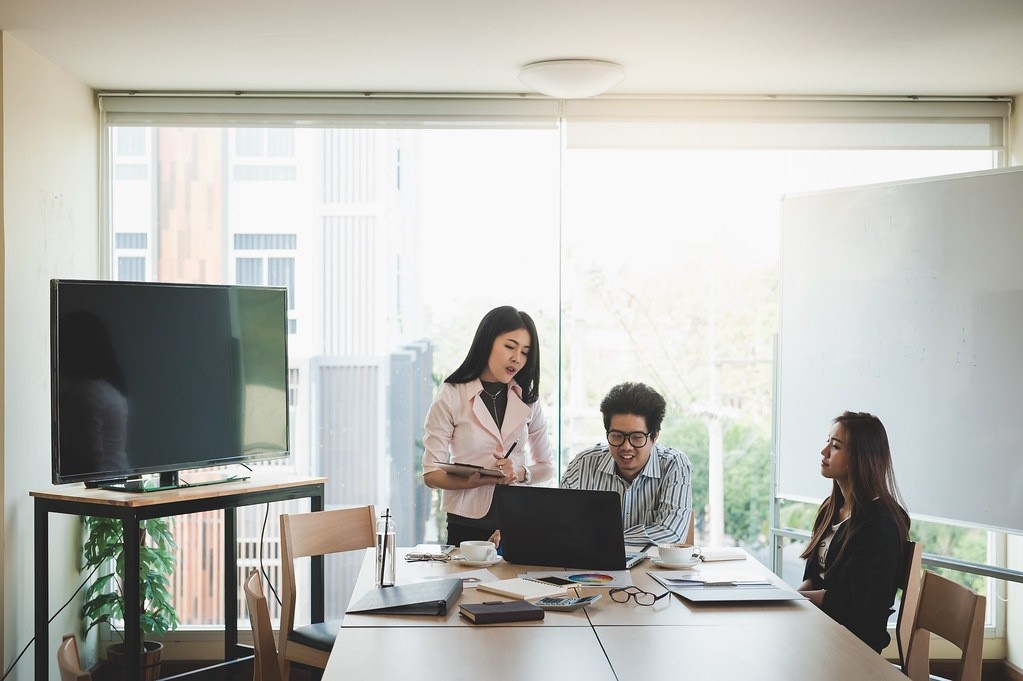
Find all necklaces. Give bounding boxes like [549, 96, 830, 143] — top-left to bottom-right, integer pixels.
[484, 385, 504, 399]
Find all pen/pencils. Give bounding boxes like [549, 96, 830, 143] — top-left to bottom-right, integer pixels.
[640, 543, 652, 552]
[504, 439, 519, 459]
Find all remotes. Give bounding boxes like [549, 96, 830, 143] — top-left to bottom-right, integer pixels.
[85, 474, 143, 489]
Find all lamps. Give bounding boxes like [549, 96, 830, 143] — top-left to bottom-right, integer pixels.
[520, 59, 625, 100]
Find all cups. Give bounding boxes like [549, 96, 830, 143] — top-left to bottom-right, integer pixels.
[460, 541, 497, 561]
[658, 543, 701, 563]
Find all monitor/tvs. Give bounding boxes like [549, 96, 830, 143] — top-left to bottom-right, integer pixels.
[49, 278, 290, 492]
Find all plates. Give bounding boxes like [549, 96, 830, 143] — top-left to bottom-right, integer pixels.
[650, 556, 702, 569]
[453, 554, 503, 568]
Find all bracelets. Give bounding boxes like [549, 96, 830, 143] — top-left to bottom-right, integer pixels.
[520, 465, 531, 485]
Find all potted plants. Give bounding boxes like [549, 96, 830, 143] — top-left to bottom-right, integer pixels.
[78, 515, 183, 681]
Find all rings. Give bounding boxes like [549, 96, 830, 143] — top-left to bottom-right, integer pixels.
[500, 466, 502, 470]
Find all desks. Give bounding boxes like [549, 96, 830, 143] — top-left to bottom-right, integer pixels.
[321, 546, 911, 681]
[29, 474, 328, 681]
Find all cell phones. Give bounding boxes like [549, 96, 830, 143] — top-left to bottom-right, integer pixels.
[537, 576, 582, 587]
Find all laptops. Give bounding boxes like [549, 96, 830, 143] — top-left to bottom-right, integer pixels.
[494, 485, 646, 571]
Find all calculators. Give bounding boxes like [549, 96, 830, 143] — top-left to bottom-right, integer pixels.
[533, 594, 603, 613]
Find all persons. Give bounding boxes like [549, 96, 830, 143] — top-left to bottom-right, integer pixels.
[422, 306, 555, 548]
[797, 411, 911, 656]
[488, 382, 692, 549]
[59, 300, 129, 475]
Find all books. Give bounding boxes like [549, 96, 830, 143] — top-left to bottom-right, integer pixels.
[477, 577, 567, 600]
[458, 601, 545, 625]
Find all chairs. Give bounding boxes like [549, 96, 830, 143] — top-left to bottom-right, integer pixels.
[280, 504, 377, 681]
[244, 570, 283, 681]
[880, 542, 986, 681]
[58, 634, 91, 681]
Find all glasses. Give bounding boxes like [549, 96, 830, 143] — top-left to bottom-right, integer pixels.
[608, 585, 670, 606]
[405, 552, 451, 562]
[607, 430, 653, 448]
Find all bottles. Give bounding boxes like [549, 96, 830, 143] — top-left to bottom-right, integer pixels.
[376, 510, 396, 587]
